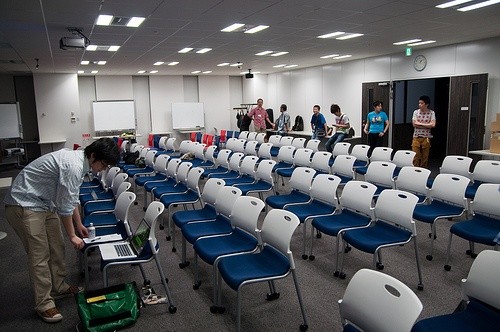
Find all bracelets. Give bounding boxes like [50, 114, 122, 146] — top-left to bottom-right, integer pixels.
[382, 131, 385, 133]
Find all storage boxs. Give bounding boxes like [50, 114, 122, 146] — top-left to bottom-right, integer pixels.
[490, 138, 500, 154]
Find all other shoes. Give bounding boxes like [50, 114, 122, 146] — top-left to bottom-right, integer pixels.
[0, 231, 8, 240]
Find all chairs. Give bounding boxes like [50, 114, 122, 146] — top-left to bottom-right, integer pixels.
[79, 131, 500, 332]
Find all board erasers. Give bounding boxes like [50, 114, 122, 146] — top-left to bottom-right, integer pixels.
[196, 126, 200, 127]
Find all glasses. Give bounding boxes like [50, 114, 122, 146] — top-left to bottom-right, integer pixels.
[100, 159, 111, 170]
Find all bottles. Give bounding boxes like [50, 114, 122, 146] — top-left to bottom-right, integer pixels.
[87, 223, 95, 239]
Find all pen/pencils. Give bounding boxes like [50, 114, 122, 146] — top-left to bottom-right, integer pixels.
[91, 237, 101, 242]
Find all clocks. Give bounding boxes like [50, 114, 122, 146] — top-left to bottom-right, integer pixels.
[414, 55, 426, 71]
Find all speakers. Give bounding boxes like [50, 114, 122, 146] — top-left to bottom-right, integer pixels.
[245, 74, 254, 78]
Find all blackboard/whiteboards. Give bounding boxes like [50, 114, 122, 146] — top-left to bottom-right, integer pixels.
[93, 100, 136, 131]
[0, 102, 23, 140]
[172, 103, 204, 129]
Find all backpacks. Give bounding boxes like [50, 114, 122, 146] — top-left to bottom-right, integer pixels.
[292, 116, 304, 131]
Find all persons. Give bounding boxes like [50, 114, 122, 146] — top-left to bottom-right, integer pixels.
[311, 105, 329, 140]
[364, 101, 389, 152]
[410, 96, 436, 169]
[275, 105, 292, 135]
[247, 99, 274, 138]
[325, 105, 351, 153]
[2, 138, 120, 323]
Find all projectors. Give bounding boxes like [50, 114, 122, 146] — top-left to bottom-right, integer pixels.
[4, 148, 25, 154]
[59, 37, 85, 51]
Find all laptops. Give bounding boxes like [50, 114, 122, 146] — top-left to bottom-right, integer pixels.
[96, 216, 151, 262]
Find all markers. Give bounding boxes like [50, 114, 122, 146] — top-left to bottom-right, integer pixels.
[129, 129, 134, 130]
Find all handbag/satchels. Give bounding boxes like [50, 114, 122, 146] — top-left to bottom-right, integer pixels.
[74, 280, 146, 332]
[316, 128, 326, 137]
[346, 127, 355, 138]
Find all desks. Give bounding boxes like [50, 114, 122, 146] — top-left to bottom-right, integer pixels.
[266, 129, 362, 143]
[469, 150, 500, 160]
[38, 140, 66, 152]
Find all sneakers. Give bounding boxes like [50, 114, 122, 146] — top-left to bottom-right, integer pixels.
[39, 307, 63, 322]
[51, 286, 83, 300]
